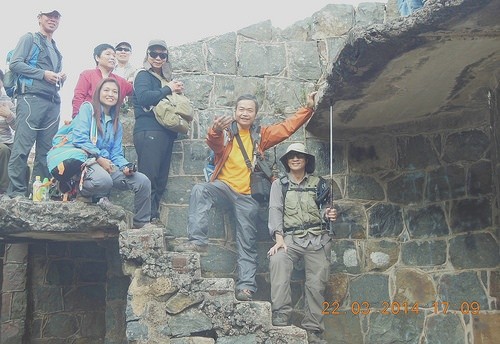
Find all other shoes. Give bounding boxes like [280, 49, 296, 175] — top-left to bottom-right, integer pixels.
[153, 217, 166, 229]
[132, 222, 157, 228]
[76, 191, 91, 202]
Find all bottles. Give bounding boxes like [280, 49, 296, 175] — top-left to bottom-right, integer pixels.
[33, 176, 42, 202]
[43, 178, 50, 201]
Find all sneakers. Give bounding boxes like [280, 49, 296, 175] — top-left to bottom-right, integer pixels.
[175, 242, 208, 255]
[308, 332, 326, 344]
[237, 289, 252, 301]
[272, 312, 288, 325]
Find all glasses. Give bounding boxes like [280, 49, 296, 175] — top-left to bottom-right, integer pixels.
[116, 48, 130, 51]
[286, 152, 305, 159]
[148, 51, 168, 59]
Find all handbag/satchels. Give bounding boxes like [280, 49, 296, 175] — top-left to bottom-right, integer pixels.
[250, 172, 271, 202]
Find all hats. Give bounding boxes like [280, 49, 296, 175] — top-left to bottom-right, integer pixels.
[148, 39, 167, 51]
[280, 143, 315, 172]
[37, 7, 62, 17]
[114, 40, 132, 48]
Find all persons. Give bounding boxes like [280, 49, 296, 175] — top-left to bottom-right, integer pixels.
[72, 44, 135, 121]
[267, 143, 337, 334]
[63, 77, 152, 228]
[0, 69, 18, 192]
[7, 7, 67, 198]
[133, 40, 185, 228]
[113, 41, 137, 81]
[184, 90, 318, 301]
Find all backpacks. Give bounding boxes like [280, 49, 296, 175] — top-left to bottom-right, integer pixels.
[133, 68, 194, 134]
[1, 31, 41, 95]
[47, 101, 98, 180]
